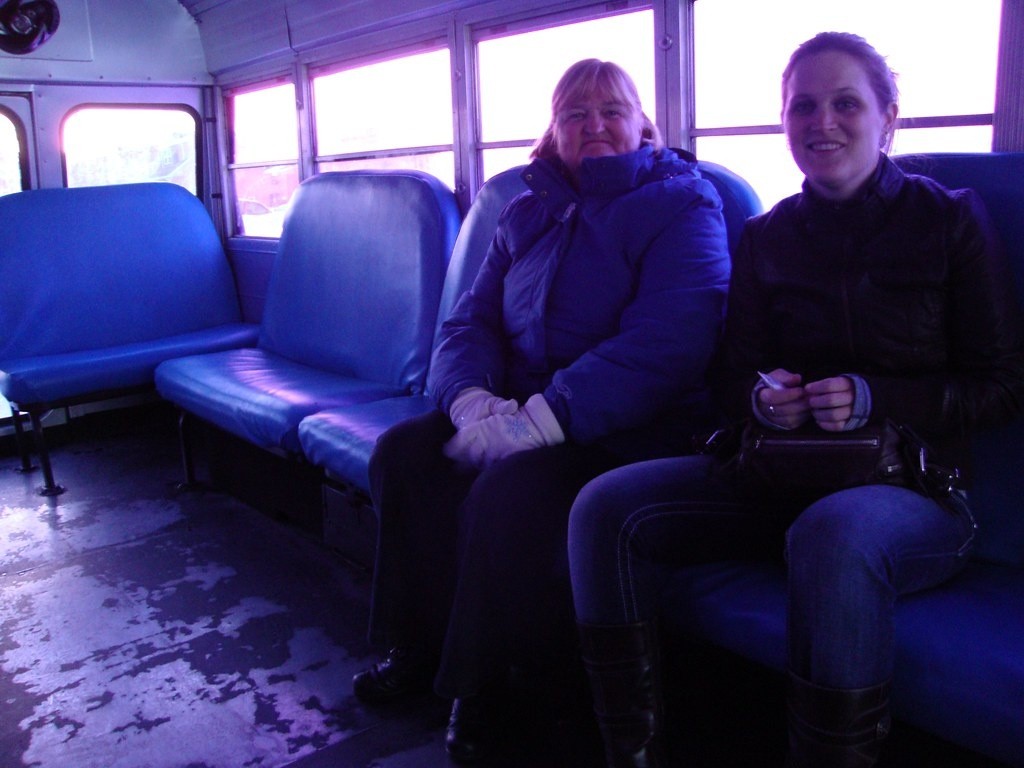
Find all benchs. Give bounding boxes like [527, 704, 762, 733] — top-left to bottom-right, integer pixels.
[153, 170, 460, 451]
[693, 150, 1024, 767]
[295, 164, 749, 563]
[0, 183, 259, 495]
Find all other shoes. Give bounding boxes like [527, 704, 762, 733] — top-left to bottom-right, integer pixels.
[351, 646, 441, 699]
[443, 702, 483, 757]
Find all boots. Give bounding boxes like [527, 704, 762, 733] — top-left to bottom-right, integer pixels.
[575, 615, 661, 768]
[773, 664, 895, 768]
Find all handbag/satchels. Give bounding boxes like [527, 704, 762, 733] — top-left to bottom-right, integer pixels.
[734, 416, 976, 517]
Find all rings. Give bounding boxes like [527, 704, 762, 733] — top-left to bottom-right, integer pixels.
[769, 406, 774, 415]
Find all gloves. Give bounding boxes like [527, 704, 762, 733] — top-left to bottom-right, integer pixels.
[449, 386, 518, 431]
[442, 393, 566, 465]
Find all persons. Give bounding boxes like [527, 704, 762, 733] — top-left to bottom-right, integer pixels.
[352, 61, 731, 761]
[567, 32, 1024, 768]
